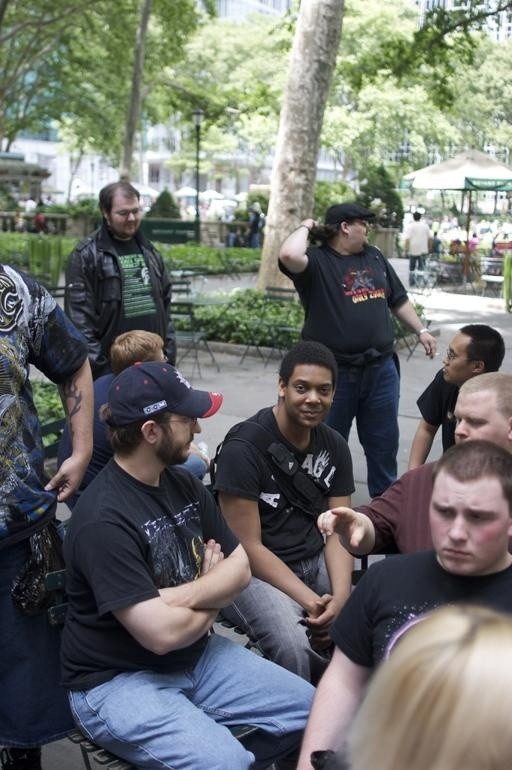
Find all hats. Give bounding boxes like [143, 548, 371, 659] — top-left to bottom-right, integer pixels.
[324, 203, 377, 225]
[105, 360, 225, 428]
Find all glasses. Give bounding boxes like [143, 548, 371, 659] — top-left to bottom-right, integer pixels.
[446, 349, 473, 364]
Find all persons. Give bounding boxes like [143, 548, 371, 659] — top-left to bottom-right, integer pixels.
[247, 202, 264, 247]
[278, 202, 438, 500]
[64, 182, 176, 369]
[15, 193, 60, 234]
[56, 323, 512, 770]
[1, 265, 95, 770]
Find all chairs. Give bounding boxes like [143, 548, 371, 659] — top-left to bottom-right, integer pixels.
[40, 418, 66, 458]
[480, 256, 505, 298]
[166, 249, 297, 379]
[44, 568, 260, 769]
[204, 441, 367, 660]
[391, 313, 432, 362]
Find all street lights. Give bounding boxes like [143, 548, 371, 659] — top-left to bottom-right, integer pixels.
[189, 107, 204, 245]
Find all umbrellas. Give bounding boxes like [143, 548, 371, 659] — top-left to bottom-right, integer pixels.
[402, 148, 512, 286]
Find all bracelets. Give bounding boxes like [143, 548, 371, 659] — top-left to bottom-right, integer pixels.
[417, 327, 428, 335]
